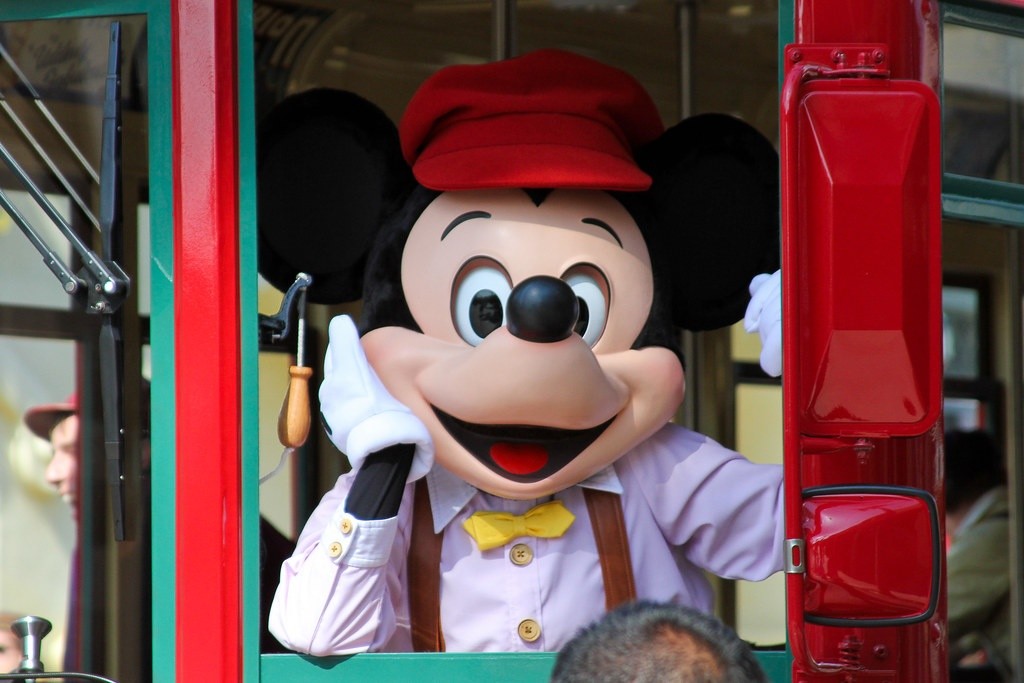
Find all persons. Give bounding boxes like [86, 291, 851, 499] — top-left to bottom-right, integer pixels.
[0, 391, 1013, 683]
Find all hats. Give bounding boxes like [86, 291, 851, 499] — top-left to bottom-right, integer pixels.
[24, 391, 78, 441]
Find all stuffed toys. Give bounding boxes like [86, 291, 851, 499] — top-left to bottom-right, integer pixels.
[256, 50, 783, 652]
